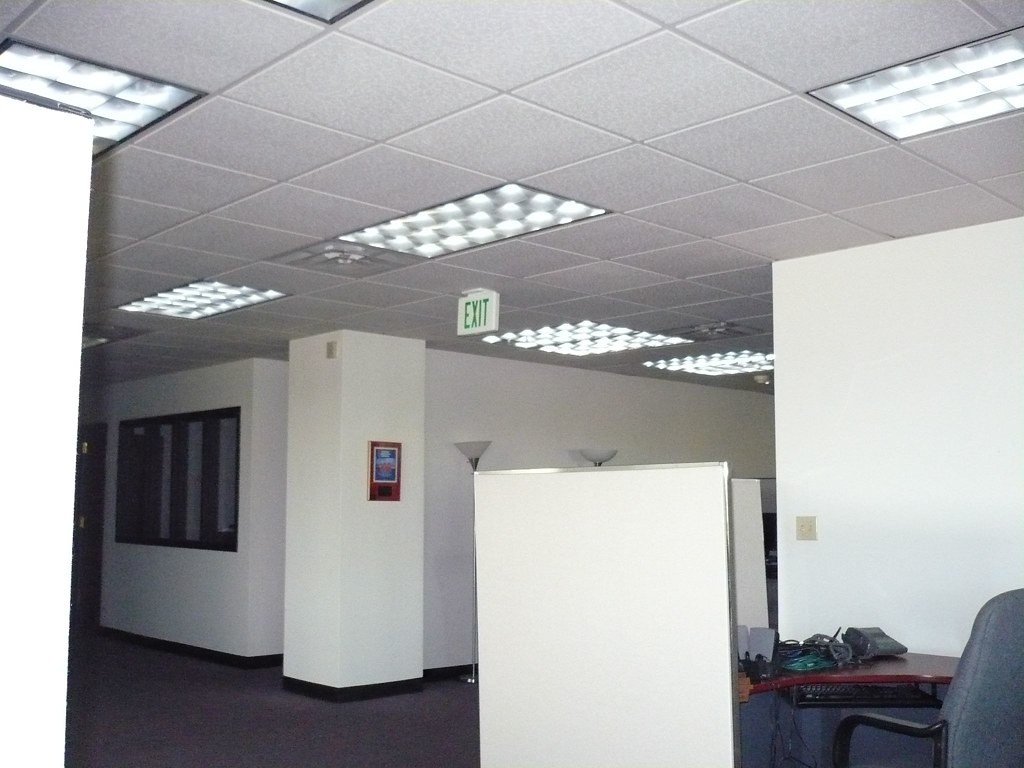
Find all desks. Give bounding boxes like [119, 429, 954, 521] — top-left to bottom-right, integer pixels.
[742, 651, 960, 709]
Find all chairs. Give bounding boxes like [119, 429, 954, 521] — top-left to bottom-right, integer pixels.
[832, 588, 1024, 768]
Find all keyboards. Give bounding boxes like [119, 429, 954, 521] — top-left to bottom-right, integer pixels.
[800, 683, 924, 703]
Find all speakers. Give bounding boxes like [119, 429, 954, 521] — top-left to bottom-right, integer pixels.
[748, 627, 780, 665]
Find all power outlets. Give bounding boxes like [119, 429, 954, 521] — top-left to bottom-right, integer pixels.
[796, 516, 818, 541]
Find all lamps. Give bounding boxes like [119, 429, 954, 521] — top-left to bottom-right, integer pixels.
[580, 449, 618, 466]
[454, 441, 492, 683]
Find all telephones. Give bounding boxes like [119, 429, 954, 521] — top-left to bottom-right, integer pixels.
[842, 626, 909, 661]
